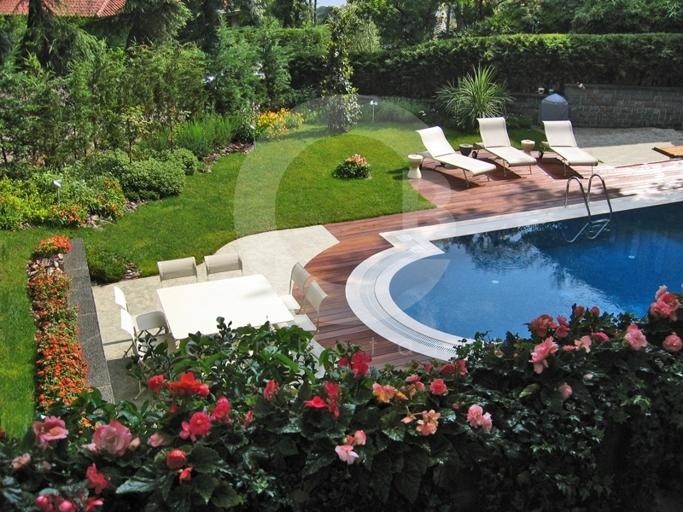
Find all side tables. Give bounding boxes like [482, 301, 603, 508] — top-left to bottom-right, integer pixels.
[521, 139, 535, 156]
[458, 144, 473, 156]
[407, 155, 422, 178]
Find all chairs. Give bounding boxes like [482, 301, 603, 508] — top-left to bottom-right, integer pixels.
[416, 126, 496, 188]
[476, 117, 537, 177]
[114, 253, 328, 401]
[540, 120, 598, 176]
